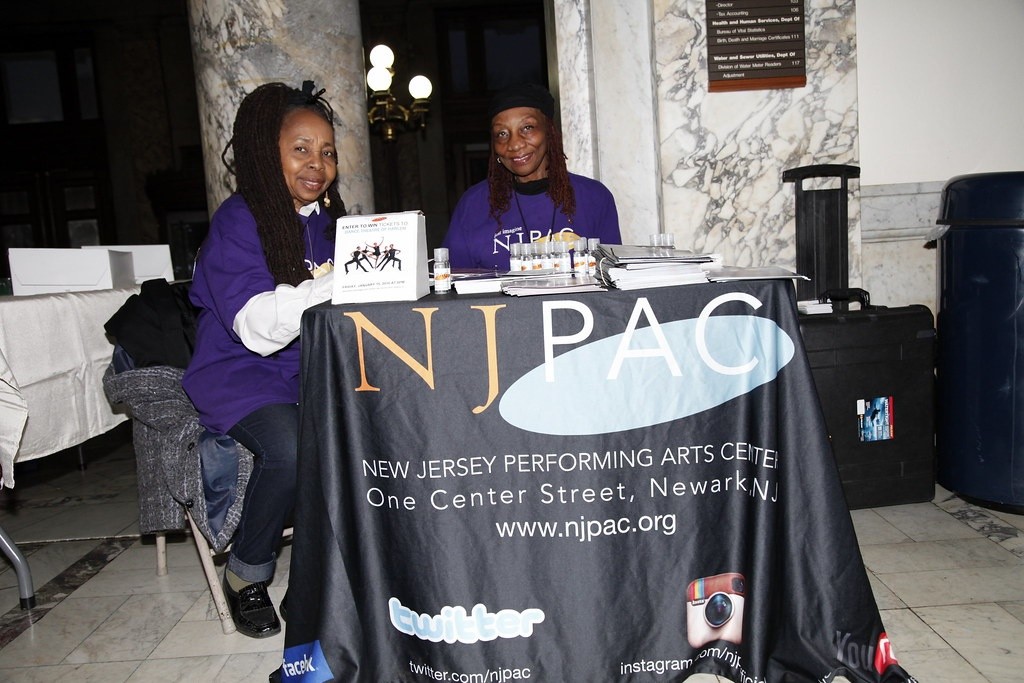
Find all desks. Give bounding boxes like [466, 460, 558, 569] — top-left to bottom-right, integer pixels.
[0, 279, 193, 610]
[270, 287, 919, 683]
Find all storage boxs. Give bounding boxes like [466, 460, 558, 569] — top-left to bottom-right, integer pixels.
[8, 248, 135, 296]
[331, 210, 430, 305]
[81, 245, 186, 284]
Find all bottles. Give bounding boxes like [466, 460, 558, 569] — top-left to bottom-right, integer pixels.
[433, 248, 451, 295]
[509, 237, 601, 276]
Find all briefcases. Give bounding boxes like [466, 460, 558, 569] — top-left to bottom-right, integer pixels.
[799, 288, 938, 510]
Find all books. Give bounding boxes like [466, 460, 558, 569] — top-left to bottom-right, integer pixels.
[452, 245, 810, 297]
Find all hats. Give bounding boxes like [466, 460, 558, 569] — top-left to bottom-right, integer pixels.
[488, 71, 553, 129]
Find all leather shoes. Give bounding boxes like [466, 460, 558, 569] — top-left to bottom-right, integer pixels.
[280, 586, 290, 621]
[224, 561, 281, 639]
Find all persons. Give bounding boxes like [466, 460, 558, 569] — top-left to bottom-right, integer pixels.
[181, 79, 345, 639]
[345, 237, 401, 275]
[442, 78, 622, 272]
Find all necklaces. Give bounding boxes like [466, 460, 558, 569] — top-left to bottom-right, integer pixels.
[513, 179, 556, 243]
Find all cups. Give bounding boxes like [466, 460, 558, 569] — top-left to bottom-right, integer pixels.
[649, 232, 674, 247]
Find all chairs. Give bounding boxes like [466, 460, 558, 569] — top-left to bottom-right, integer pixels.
[102, 277, 294, 635]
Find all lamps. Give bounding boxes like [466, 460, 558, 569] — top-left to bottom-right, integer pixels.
[366, 44, 433, 141]
